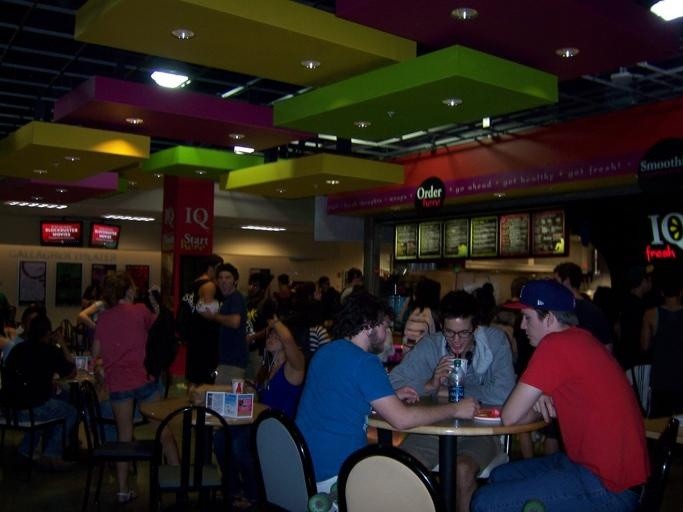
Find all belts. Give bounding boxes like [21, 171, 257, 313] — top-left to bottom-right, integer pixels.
[628, 485, 645, 496]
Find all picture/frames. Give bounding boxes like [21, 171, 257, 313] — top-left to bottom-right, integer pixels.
[91, 263, 117, 285]
[125, 265, 149, 290]
[55, 262, 82, 307]
[18, 260, 47, 306]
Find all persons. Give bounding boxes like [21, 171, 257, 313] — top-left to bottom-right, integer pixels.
[91, 273, 180, 506]
[388, 290, 516, 512]
[295, 291, 481, 511]
[198, 263, 247, 386]
[0, 303, 46, 367]
[0, 292, 17, 348]
[0, 303, 18, 361]
[242, 268, 363, 326]
[612, 267, 654, 419]
[185, 253, 223, 385]
[486, 276, 562, 457]
[639, 272, 683, 419]
[551, 262, 595, 321]
[0, 313, 77, 469]
[211, 312, 305, 512]
[468, 279, 652, 511]
[80, 283, 100, 308]
[77, 276, 114, 332]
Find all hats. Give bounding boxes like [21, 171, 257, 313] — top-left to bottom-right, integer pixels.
[500, 280, 576, 311]
[559, 263, 583, 282]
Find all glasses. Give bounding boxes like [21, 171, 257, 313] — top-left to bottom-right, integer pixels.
[443, 325, 474, 338]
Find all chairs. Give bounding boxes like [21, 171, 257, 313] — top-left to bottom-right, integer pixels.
[633, 417, 683, 511]
[78, 380, 162, 512]
[0, 365, 67, 481]
[337, 444, 446, 512]
[150, 406, 234, 512]
[251, 409, 317, 512]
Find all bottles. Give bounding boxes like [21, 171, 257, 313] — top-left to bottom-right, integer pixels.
[447, 358, 466, 405]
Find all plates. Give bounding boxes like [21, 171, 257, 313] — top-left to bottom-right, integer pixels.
[474, 410, 502, 424]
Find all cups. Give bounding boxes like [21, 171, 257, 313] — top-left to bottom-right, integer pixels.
[445, 358, 468, 381]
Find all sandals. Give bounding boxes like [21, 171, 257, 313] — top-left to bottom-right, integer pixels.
[210, 492, 257, 510]
[117, 489, 137, 505]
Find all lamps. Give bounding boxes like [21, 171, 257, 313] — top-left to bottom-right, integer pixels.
[649, 0, 683, 24]
[150, 69, 192, 92]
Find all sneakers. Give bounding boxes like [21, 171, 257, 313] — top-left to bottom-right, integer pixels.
[41, 453, 65, 465]
[19, 449, 40, 461]
